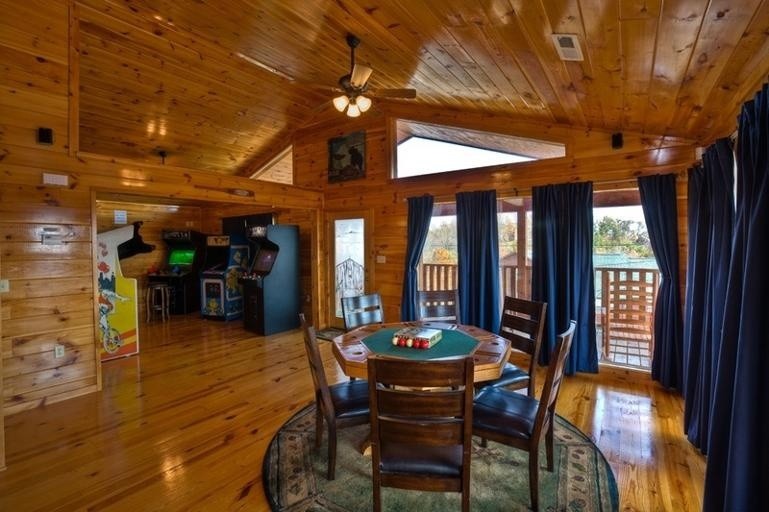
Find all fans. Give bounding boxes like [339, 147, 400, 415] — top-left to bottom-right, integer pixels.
[284, 32, 417, 131]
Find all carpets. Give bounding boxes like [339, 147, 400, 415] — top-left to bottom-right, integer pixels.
[262, 401, 620, 511]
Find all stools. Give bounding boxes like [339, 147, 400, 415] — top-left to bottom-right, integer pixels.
[145, 281, 170, 324]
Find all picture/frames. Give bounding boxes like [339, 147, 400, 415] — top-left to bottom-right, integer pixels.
[327, 130, 366, 184]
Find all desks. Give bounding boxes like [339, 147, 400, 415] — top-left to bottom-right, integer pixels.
[331, 321, 512, 456]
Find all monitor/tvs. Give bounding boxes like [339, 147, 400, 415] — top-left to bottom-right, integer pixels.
[252, 249, 277, 274]
[167, 249, 196, 265]
[209, 249, 229, 271]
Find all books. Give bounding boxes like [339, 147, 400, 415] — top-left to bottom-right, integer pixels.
[391, 325, 444, 349]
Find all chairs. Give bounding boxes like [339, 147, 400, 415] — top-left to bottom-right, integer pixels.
[299, 314, 386, 480]
[367, 355, 472, 512]
[416, 291, 460, 325]
[422, 264, 661, 363]
[473, 320, 575, 510]
[472, 295, 547, 398]
[342, 293, 385, 381]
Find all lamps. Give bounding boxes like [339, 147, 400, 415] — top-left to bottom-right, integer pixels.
[332, 64, 373, 118]
